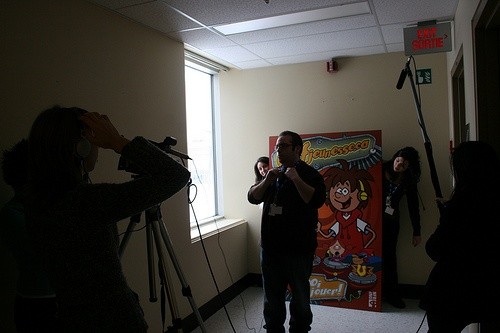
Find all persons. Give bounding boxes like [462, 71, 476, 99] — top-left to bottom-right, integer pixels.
[421, 140, 500, 333]
[248, 131, 326, 333]
[0, 107, 190, 333]
[254, 157, 269, 183]
[381, 147, 422, 309]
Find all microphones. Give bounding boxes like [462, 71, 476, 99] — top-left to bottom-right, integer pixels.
[396, 56, 412, 89]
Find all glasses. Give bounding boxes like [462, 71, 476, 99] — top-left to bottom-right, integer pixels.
[274, 143, 294, 149]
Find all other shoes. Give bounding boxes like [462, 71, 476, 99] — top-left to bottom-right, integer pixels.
[385, 295, 407, 309]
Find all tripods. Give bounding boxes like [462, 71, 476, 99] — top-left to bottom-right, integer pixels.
[119, 205, 206, 333]
